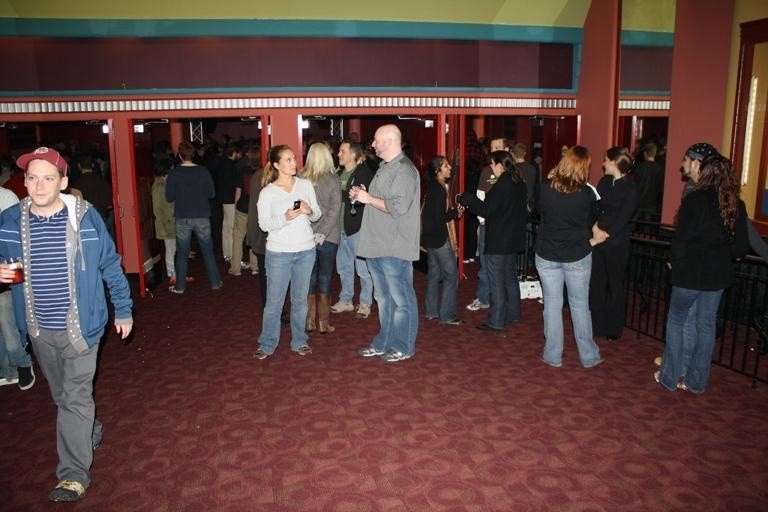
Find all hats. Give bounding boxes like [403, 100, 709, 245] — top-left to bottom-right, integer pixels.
[685, 143, 718, 161]
[16, 147, 68, 177]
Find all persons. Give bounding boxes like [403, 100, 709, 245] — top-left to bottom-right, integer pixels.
[0, 158, 36, 390]
[245, 125, 422, 361]
[60, 135, 263, 293]
[654, 143, 749, 392]
[0, 147, 134, 501]
[419, 137, 670, 368]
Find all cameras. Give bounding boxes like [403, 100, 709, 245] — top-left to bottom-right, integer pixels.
[293, 200, 300, 210]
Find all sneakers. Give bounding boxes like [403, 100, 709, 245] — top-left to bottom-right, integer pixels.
[17, 364, 36, 391]
[47, 480, 88, 504]
[0, 377, 19, 386]
[168, 251, 260, 294]
[653, 370, 691, 391]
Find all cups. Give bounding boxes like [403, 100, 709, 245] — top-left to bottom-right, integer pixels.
[5, 257, 26, 286]
